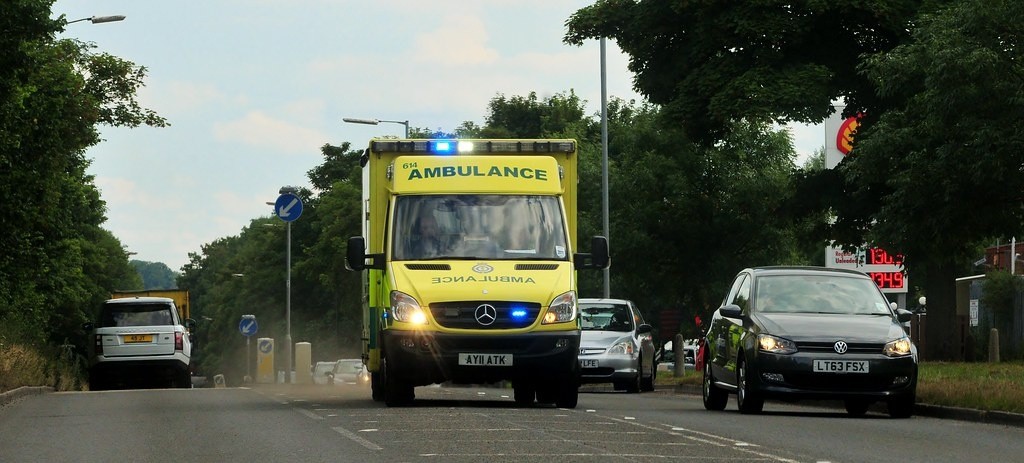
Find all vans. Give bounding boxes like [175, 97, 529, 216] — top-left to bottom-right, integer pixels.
[84, 295, 196, 391]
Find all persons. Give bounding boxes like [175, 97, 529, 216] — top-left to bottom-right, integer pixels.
[404, 213, 449, 259]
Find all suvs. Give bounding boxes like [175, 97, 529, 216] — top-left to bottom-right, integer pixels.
[576, 295, 659, 392]
[700, 264, 919, 417]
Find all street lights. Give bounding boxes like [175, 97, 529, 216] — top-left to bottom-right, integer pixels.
[341, 117, 410, 138]
[262, 191, 294, 386]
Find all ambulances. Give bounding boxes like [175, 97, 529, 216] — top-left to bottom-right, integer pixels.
[341, 130, 609, 410]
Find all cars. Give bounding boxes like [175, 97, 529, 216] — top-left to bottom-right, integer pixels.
[276, 358, 371, 388]
[656, 338, 699, 373]
